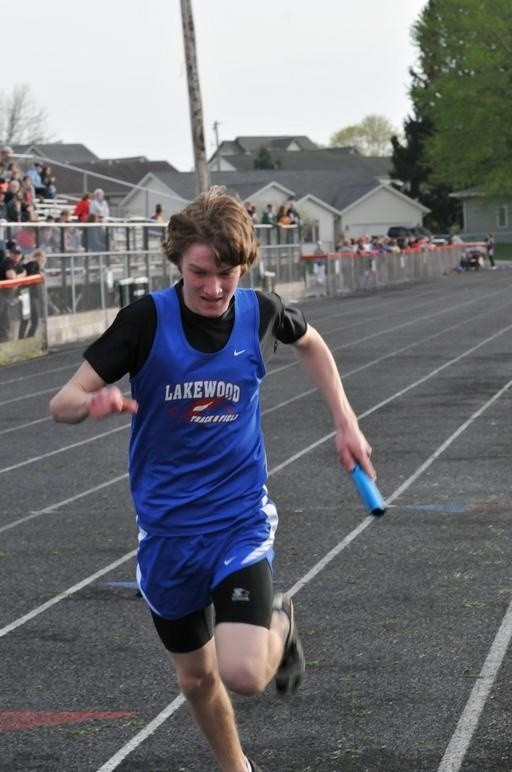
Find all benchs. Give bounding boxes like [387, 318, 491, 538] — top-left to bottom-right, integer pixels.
[0, 198, 166, 278]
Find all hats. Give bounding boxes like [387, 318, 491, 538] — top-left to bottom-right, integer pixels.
[10, 245, 21, 254]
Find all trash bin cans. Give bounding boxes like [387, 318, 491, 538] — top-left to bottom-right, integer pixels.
[118, 277, 150, 308]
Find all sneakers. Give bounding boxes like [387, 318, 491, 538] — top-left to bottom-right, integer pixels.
[274, 594, 305, 698]
[244, 756, 262, 772]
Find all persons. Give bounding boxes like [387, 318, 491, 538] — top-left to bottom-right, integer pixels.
[275, 205, 292, 224]
[286, 202, 301, 225]
[310, 241, 326, 282]
[149, 203, 165, 223]
[483, 233, 498, 270]
[44, 181, 376, 770]
[448, 231, 464, 244]
[248, 206, 259, 225]
[329, 234, 436, 255]
[243, 202, 252, 215]
[261, 204, 273, 225]
[2, 135, 115, 345]
